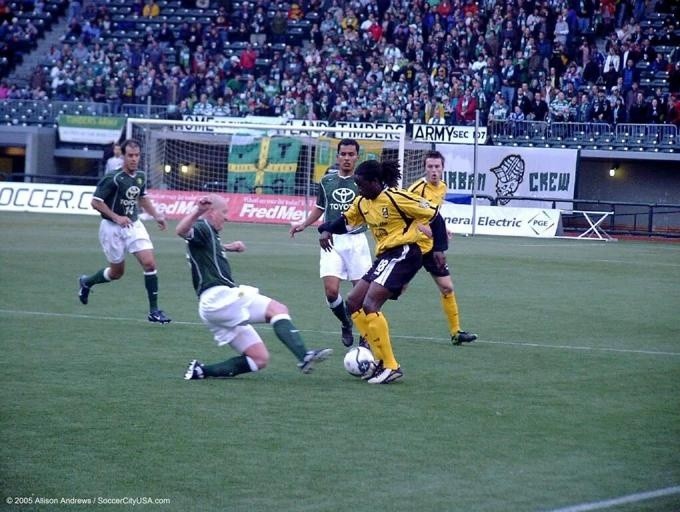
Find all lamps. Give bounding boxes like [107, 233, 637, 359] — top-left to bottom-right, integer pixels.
[181, 162, 191, 173]
[610, 166, 619, 176]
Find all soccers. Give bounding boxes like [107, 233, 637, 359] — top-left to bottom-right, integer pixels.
[344, 344, 378, 379]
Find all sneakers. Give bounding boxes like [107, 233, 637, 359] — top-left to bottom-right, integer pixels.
[362, 361, 403, 384]
[359, 335, 370, 350]
[342, 322, 354, 346]
[451, 330, 477, 346]
[148, 311, 171, 323]
[185, 359, 204, 380]
[78, 275, 90, 305]
[298, 349, 332, 373]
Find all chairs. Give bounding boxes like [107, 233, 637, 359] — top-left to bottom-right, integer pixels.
[493, 13, 680, 153]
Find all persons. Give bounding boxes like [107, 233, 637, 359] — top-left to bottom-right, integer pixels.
[290, 138, 369, 350]
[318, 159, 449, 385]
[407, 150, 478, 346]
[104, 145, 124, 175]
[175, 193, 334, 381]
[78, 139, 172, 323]
[0, 1, 680, 134]
